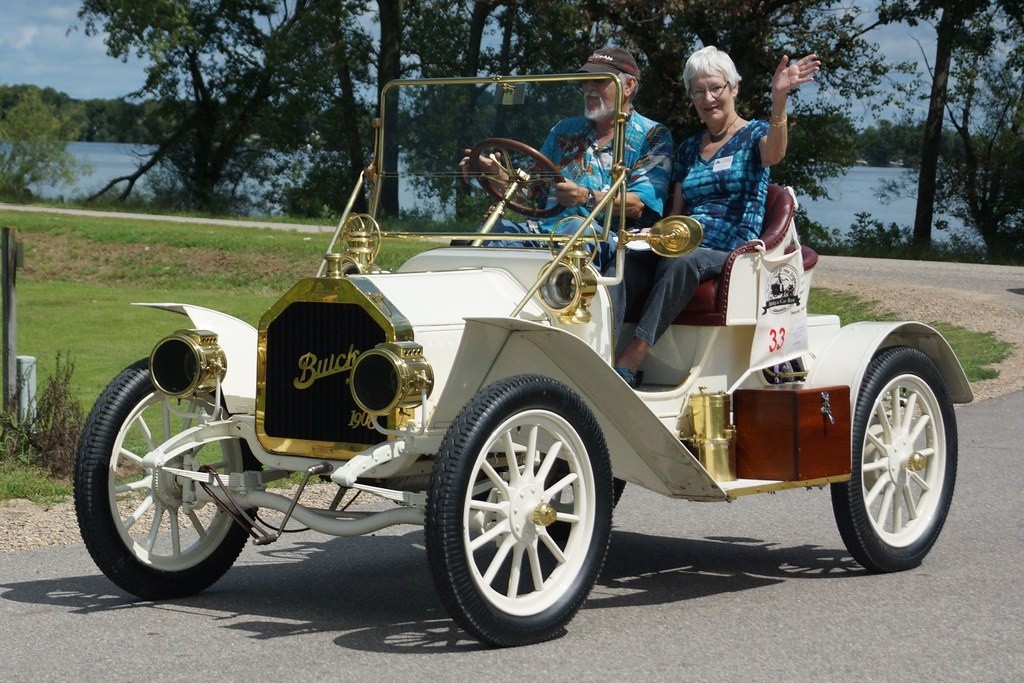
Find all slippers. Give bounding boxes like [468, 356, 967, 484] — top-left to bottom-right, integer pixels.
[613, 364, 644, 389]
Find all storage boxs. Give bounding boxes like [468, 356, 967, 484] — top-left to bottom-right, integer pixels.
[731, 384, 851, 482]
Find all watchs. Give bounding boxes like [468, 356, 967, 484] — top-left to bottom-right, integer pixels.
[583, 187, 595, 209]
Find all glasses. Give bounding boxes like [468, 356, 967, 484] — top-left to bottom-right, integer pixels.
[689, 81, 730, 99]
[582, 79, 607, 84]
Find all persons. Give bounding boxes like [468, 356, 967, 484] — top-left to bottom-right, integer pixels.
[603, 46, 821, 389]
[459, 47, 673, 275]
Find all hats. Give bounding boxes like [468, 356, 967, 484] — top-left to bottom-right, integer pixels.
[575, 47, 640, 82]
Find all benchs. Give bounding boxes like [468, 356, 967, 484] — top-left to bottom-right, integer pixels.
[625, 177, 800, 326]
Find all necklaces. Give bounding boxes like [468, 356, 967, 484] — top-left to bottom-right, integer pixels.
[706, 115, 738, 143]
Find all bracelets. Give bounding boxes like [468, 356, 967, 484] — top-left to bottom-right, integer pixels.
[770, 108, 787, 128]
